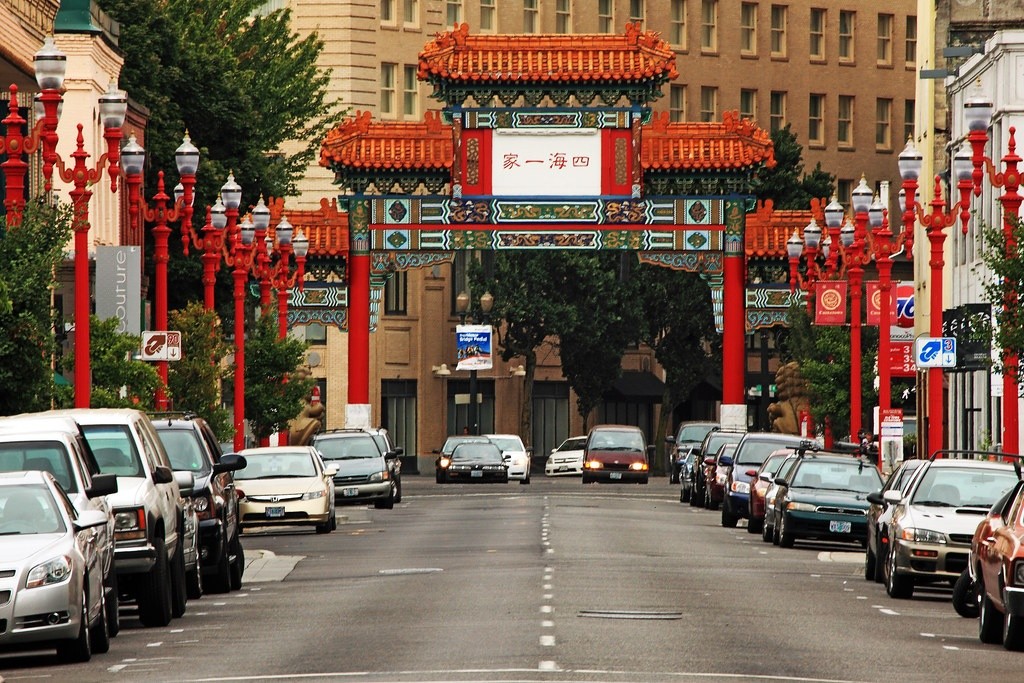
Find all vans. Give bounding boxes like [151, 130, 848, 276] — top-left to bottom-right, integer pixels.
[665, 420, 720, 484]
[582, 424, 656, 484]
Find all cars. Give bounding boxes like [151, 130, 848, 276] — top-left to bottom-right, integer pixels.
[721, 432, 885, 549]
[227, 446, 338, 535]
[704, 443, 738, 510]
[0, 470, 108, 663]
[678, 444, 702, 503]
[433, 434, 532, 484]
[864, 449, 1024, 651]
[545, 435, 588, 477]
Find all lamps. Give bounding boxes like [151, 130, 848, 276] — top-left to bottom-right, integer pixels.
[432, 364, 451, 375]
[510, 365, 525, 375]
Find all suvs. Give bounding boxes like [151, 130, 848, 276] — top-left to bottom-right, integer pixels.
[689, 426, 767, 507]
[0, 412, 118, 655]
[43, 408, 247, 629]
[310, 427, 404, 509]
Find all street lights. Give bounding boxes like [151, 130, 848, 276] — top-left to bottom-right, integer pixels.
[964, 74, 1024, 464]
[787, 171, 919, 471]
[898, 132, 973, 458]
[123, 127, 309, 452]
[457, 290, 493, 436]
[0, 32, 128, 408]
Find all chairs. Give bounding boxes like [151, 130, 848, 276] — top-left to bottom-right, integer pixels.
[802, 473, 822, 487]
[849, 476, 874, 491]
[91, 448, 129, 468]
[22, 458, 57, 479]
[929, 484, 964, 504]
